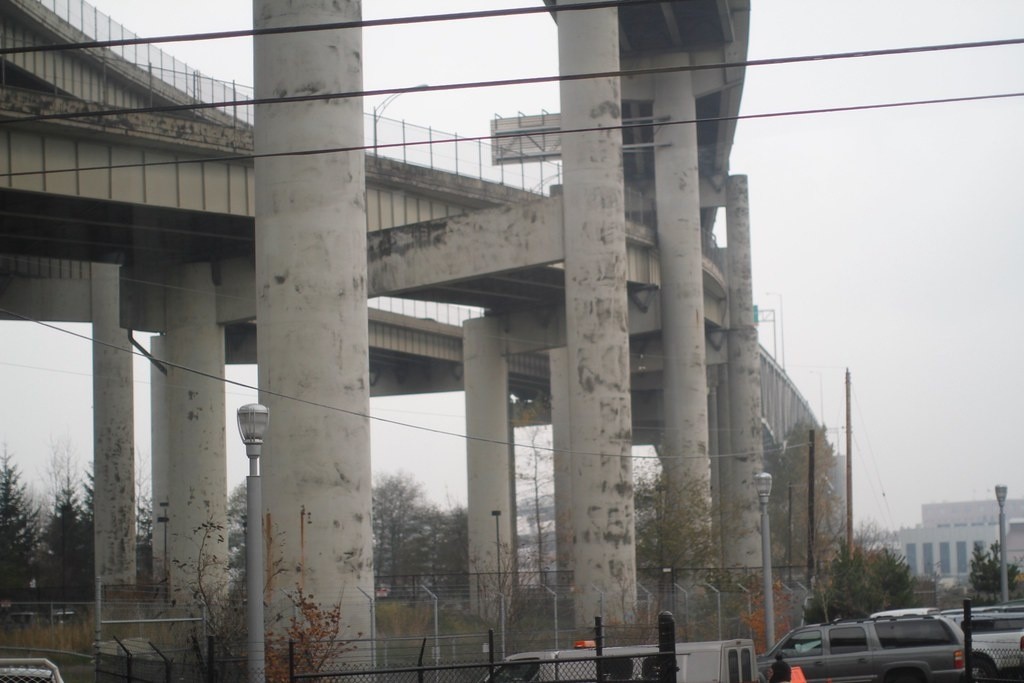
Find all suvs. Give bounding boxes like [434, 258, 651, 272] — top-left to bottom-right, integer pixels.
[755, 614, 966, 683]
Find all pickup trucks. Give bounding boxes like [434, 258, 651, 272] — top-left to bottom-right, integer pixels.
[868, 608, 1023, 681]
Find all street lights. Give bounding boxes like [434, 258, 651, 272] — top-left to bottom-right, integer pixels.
[235, 402, 270, 682]
[995, 484, 1009, 602]
[755, 472, 776, 651]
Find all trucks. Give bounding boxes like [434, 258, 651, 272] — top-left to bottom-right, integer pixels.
[477, 640, 757, 683]
